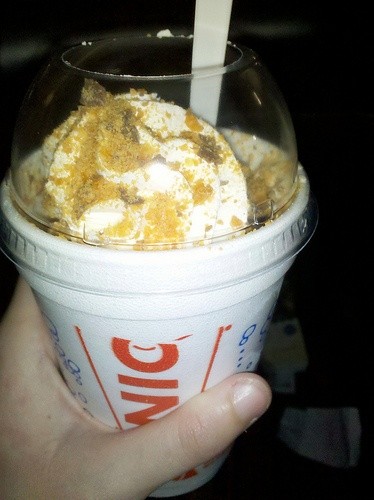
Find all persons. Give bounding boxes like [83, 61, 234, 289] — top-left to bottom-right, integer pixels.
[0, 277, 273, 500]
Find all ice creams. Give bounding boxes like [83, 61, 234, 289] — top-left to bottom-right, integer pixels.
[5, 89, 252, 251]
